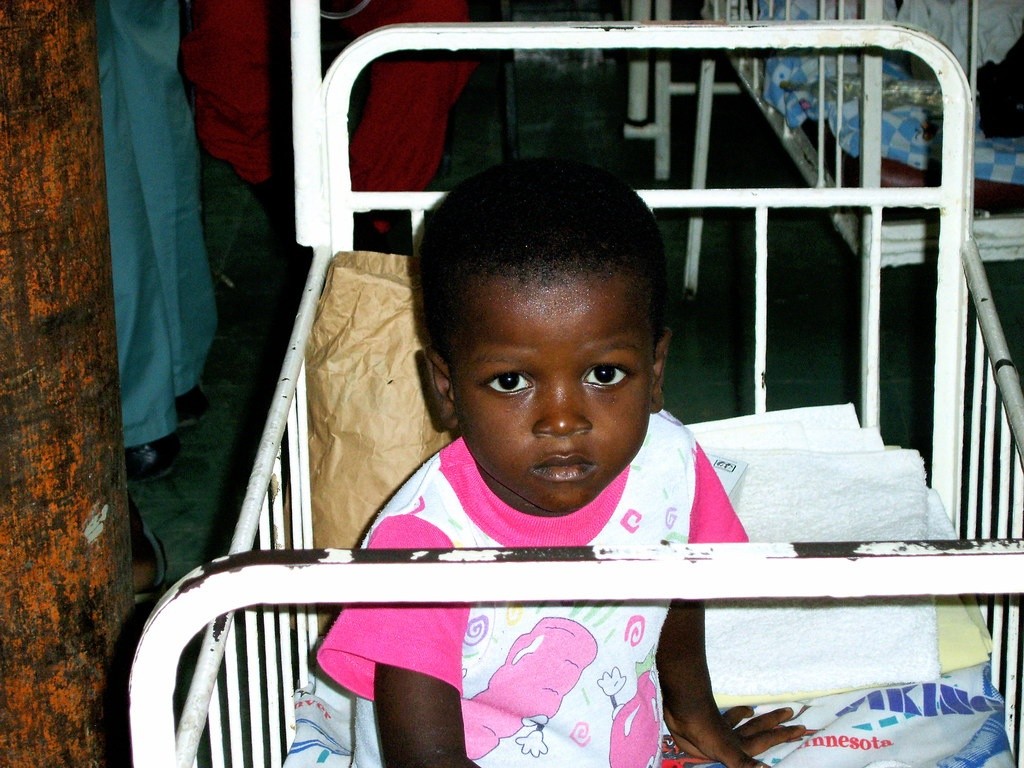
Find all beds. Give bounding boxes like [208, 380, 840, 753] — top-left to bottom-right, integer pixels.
[678, 0, 1024, 302]
[126, 21, 1024, 768]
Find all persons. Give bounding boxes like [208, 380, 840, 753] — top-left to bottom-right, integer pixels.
[180, 0, 479, 255]
[88, 0, 219, 481]
[318, 154, 807, 768]
